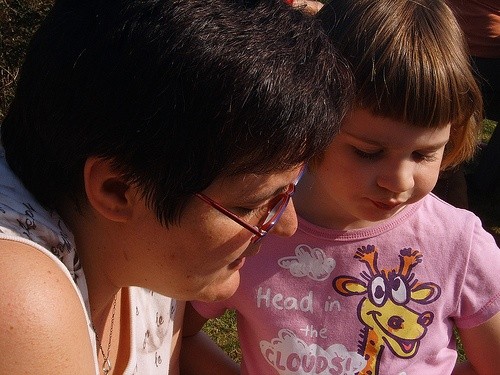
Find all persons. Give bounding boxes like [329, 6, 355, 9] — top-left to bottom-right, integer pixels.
[183, 0, 500, 375]
[0, 0, 352, 375]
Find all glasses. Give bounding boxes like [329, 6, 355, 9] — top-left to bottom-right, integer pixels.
[195, 170, 304, 244]
